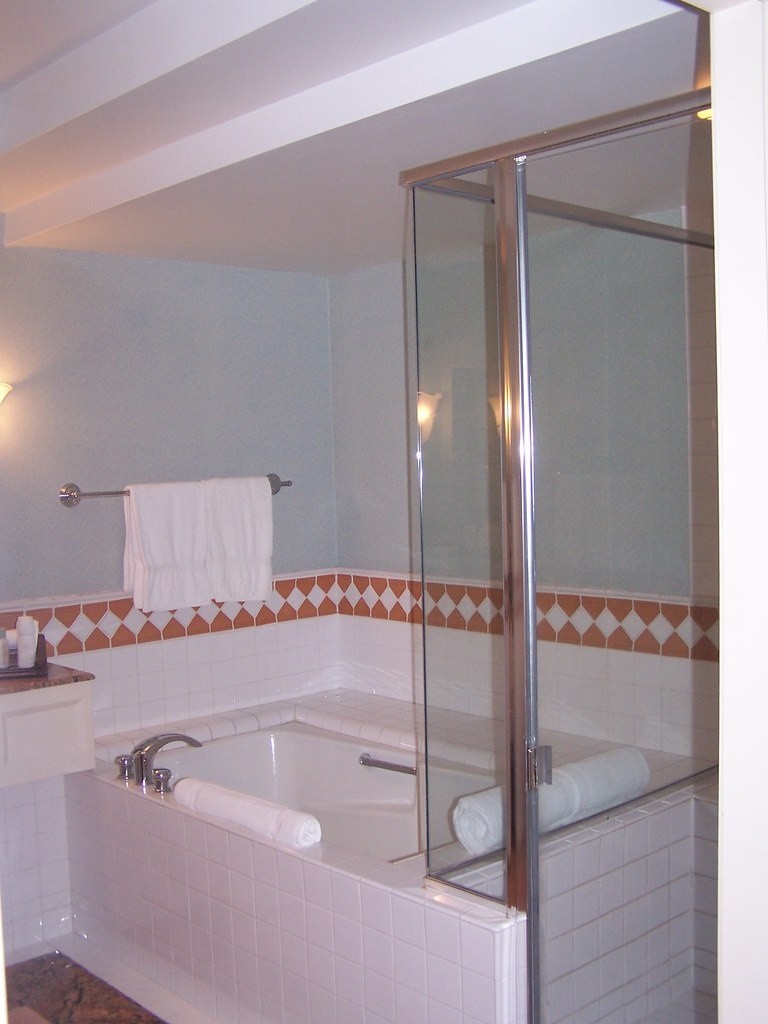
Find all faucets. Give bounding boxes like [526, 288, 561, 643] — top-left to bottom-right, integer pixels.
[129, 732, 204, 787]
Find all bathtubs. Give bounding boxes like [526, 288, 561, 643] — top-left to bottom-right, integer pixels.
[112, 717, 513, 867]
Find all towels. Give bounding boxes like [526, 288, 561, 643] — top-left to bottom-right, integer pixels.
[451, 744, 652, 858]
[121, 474, 275, 615]
[172, 776, 323, 850]
